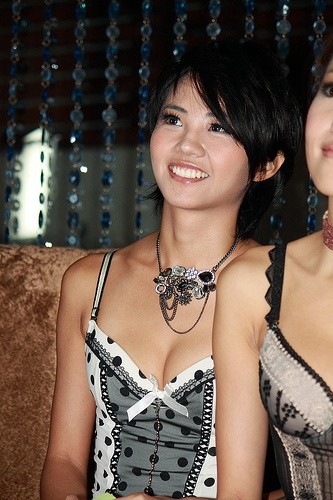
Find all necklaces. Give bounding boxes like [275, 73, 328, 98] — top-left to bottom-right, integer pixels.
[322, 210, 333, 250]
[155, 229, 241, 333]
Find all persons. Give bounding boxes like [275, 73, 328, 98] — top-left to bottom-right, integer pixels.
[39, 47, 304, 500]
[212, 36, 333, 500]
